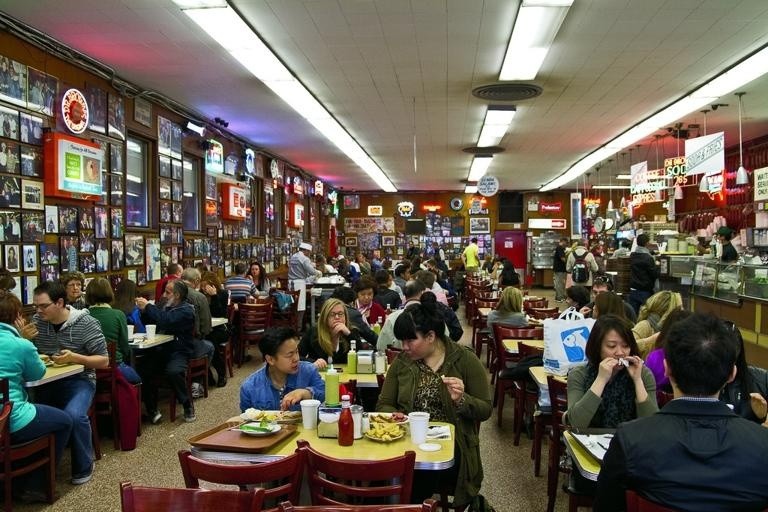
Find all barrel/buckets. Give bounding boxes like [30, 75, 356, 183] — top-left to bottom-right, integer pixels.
[606, 257, 616, 290]
[614, 256, 631, 294]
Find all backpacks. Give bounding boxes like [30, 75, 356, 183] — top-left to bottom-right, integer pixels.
[572, 249, 590, 283]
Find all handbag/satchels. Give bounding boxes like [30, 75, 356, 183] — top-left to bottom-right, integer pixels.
[469, 493, 495, 512]
[543, 306, 594, 376]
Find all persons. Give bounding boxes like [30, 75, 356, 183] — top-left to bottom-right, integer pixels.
[1, 221, 768, 512]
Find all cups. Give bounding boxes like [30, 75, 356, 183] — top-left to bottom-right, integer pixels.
[650, 248, 665, 255]
[300, 399, 324, 429]
[145, 324, 156, 340]
[678, 241, 688, 253]
[127, 325, 135, 341]
[668, 238, 679, 252]
[408, 411, 429, 443]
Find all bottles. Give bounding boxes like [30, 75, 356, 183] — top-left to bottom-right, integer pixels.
[347, 340, 387, 373]
[754, 229, 767, 246]
[472, 267, 502, 298]
[324, 363, 339, 407]
[339, 395, 370, 445]
[372, 304, 391, 335]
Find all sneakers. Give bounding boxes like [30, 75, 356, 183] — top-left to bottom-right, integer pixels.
[18, 489, 59, 503]
[235, 355, 252, 363]
[150, 407, 162, 424]
[192, 383, 204, 398]
[217, 375, 227, 386]
[71, 461, 96, 484]
[183, 403, 195, 421]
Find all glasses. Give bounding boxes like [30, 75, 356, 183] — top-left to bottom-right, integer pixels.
[328, 311, 345, 318]
[594, 276, 609, 283]
[32, 302, 53, 310]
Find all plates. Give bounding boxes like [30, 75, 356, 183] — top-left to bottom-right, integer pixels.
[366, 425, 406, 442]
[240, 410, 299, 422]
[695, 253, 715, 260]
[393, 414, 409, 424]
[44, 360, 54, 367]
[242, 422, 282, 436]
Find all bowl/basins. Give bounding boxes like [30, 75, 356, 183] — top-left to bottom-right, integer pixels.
[657, 235, 675, 242]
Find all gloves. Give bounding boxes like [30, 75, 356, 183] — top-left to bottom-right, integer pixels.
[317, 270, 322, 279]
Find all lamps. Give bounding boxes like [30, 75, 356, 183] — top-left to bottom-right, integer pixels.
[673, 121, 683, 200]
[585, 133, 662, 221]
[698, 108, 710, 194]
[734, 92, 751, 185]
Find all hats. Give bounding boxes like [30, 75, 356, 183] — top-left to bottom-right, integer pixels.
[711, 225, 732, 236]
[299, 242, 312, 250]
